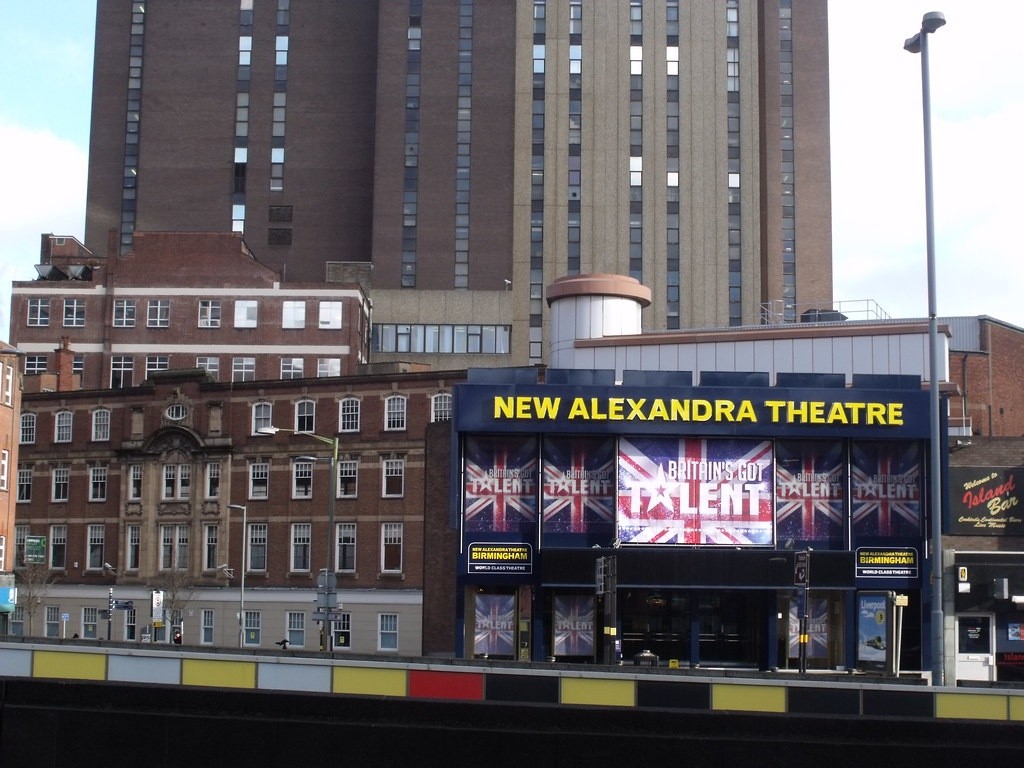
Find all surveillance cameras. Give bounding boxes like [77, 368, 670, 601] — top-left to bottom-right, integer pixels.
[217, 564, 227, 570]
[105, 563, 112, 568]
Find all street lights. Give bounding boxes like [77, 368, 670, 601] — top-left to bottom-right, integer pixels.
[900, 10, 954, 686]
[257, 426, 340, 649]
